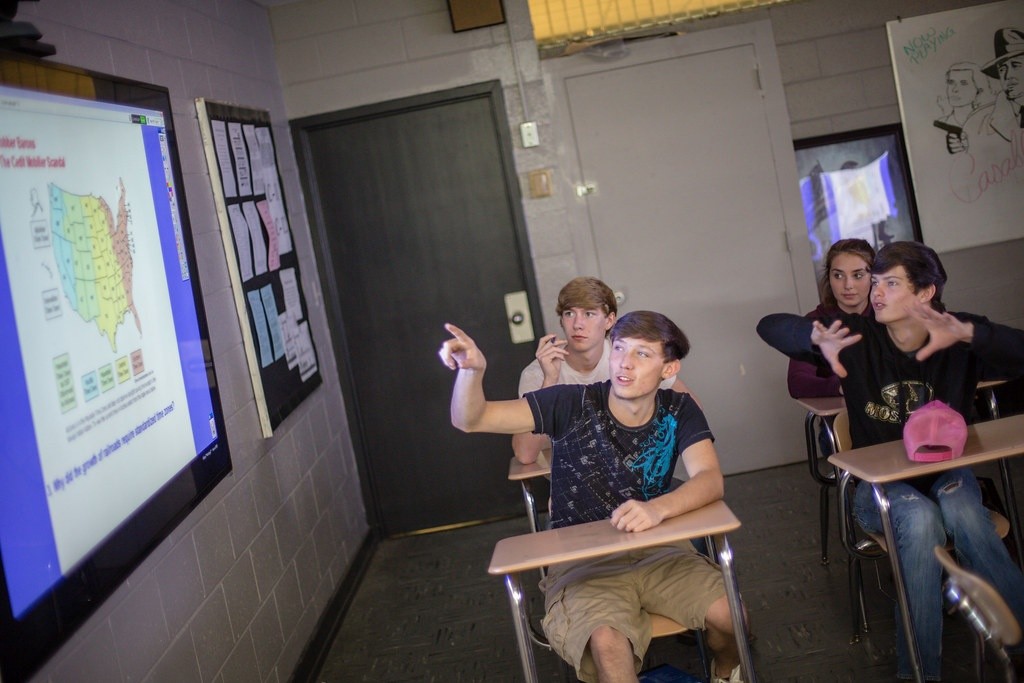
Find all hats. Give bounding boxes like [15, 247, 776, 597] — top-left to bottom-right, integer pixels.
[902, 399, 970, 463]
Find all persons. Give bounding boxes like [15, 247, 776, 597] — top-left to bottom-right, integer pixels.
[438, 311, 749, 683]
[788, 239, 876, 461]
[757, 242, 1024, 683]
[512, 278, 709, 555]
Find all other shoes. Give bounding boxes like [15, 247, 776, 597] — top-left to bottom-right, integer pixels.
[711, 658, 746, 683]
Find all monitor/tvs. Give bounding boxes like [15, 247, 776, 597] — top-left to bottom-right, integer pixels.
[0, 47, 235, 683]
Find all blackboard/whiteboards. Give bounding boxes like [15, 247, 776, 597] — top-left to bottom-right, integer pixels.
[195, 95, 324, 440]
[885, 0, 1024, 254]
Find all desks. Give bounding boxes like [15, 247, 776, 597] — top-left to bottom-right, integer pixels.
[826, 414, 1023, 683]
[794, 379, 1012, 566]
[507, 446, 553, 580]
[488, 500, 756, 683]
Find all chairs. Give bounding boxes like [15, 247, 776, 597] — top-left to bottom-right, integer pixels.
[561, 613, 712, 683]
[932, 545, 1021, 682]
[832, 413, 1024, 683]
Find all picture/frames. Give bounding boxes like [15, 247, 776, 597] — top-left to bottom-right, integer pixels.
[791, 122, 926, 286]
